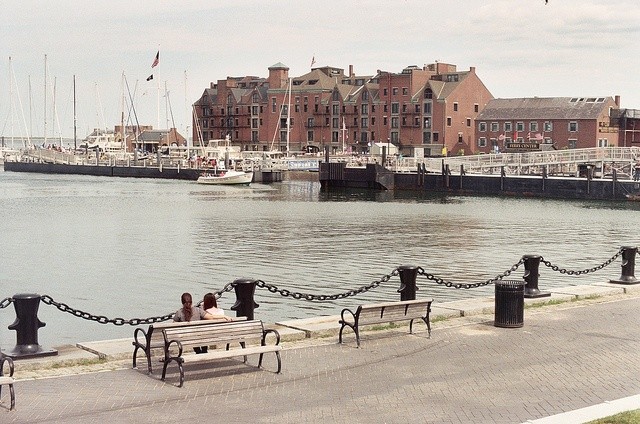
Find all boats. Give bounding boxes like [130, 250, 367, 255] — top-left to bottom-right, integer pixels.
[205, 136, 241, 153]
[196, 169, 254, 186]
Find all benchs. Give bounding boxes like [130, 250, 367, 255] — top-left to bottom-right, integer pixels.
[0, 349, 16, 410]
[133, 315, 248, 374]
[160, 321, 282, 386]
[338, 298, 434, 348]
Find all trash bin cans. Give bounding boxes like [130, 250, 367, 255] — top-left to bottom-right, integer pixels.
[495, 280, 524, 328]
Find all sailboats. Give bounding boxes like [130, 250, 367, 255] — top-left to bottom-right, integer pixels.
[268, 77, 305, 159]
[159, 70, 204, 158]
[1, 52, 150, 163]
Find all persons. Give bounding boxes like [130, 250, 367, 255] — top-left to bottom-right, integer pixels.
[172, 293, 234, 353]
[200, 293, 224, 354]
